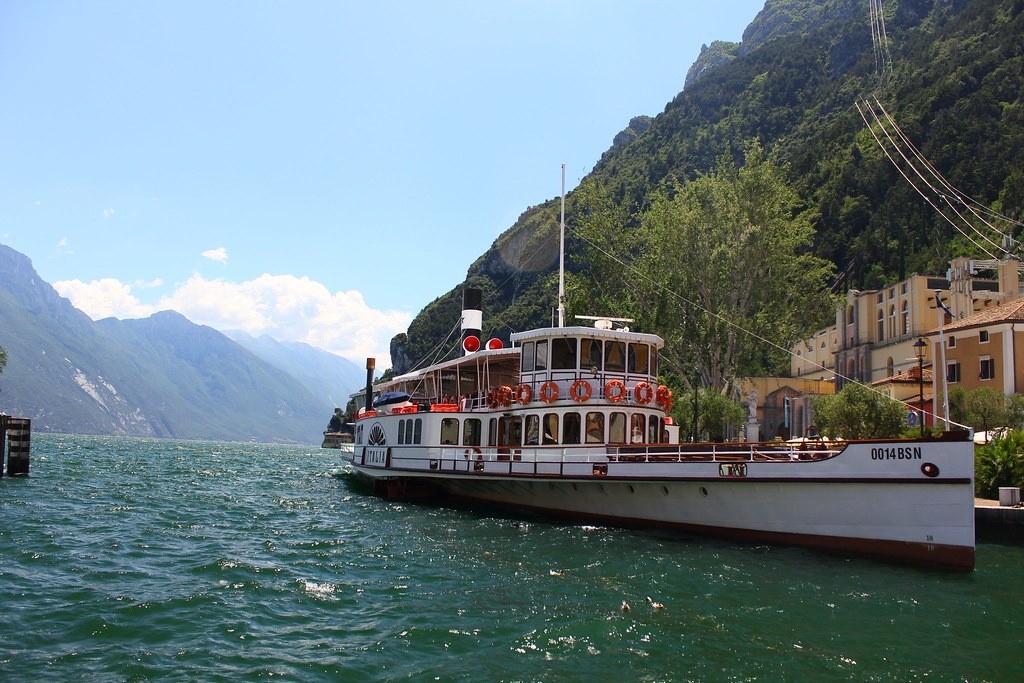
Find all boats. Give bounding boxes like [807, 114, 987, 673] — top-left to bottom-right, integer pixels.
[338, 162, 977, 574]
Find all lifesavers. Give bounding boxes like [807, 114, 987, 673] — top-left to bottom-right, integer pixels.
[487, 388, 497, 408]
[570, 381, 592, 402]
[496, 386, 513, 406]
[465, 448, 482, 460]
[448, 396, 457, 404]
[604, 380, 625, 403]
[540, 382, 559, 403]
[656, 385, 669, 406]
[635, 382, 653, 404]
[667, 391, 673, 410]
[515, 384, 532, 405]
[442, 397, 448, 404]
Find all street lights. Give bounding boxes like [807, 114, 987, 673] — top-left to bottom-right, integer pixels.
[690, 373, 700, 442]
[913, 337, 928, 442]
